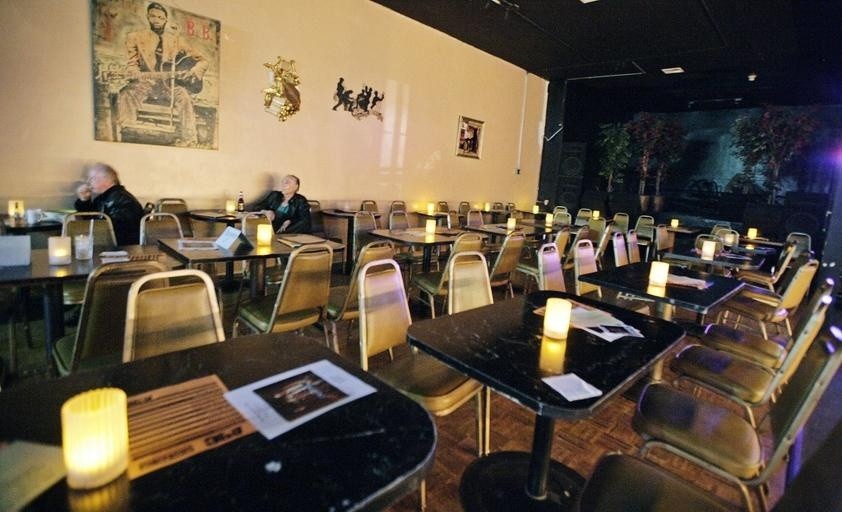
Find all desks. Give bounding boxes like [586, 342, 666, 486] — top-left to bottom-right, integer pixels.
[405, 289, 686, 511]
[1, 333, 438, 511]
[577, 261, 746, 402]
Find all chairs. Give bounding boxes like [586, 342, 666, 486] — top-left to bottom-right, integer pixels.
[0, 200, 630, 376]
[356, 258, 484, 511]
[632, 212, 841, 510]
[575, 416, 841, 510]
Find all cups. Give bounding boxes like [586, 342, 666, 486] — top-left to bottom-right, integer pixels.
[425, 233, 435, 244]
[64, 472, 132, 512]
[748, 228, 757, 240]
[700, 240, 715, 261]
[257, 246, 271, 257]
[48, 236, 72, 265]
[647, 284, 665, 298]
[27, 209, 44, 225]
[484, 203, 490, 212]
[725, 233, 733, 243]
[60, 387, 130, 490]
[592, 210, 600, 221]
[75, 235, 94, 260]
[544, 213, 553, 227]
[543, 296, 571, 342]
[532, 206, 539, 214]
[226, 199, 236, 213]
[256, 223, 272, 246]
[649, 261, 669, 286]
[425, 201, 437, 235]
[506, 218, 516, 230]
[671, 218, 679, 229]
[539, 337, 566, 374]
[8, 200, 25, 219]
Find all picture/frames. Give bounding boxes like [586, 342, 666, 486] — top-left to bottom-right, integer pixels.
[455, 116, 485, 159]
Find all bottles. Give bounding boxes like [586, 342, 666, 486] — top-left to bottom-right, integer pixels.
[13, 202, 24, 225]
[239, 191, 244, 212]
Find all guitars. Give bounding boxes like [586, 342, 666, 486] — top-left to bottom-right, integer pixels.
[98, 55, 203, 93]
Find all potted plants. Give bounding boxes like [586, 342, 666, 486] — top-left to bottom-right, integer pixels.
[629, 114, 687, 213]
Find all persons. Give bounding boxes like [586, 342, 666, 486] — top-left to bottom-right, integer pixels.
[75, 162, 144, 246]
[254, 175, 312, 234]
[459, 120, 480, 153]
[113, 3, 209, 143]
[272, 378, 339, 406]
